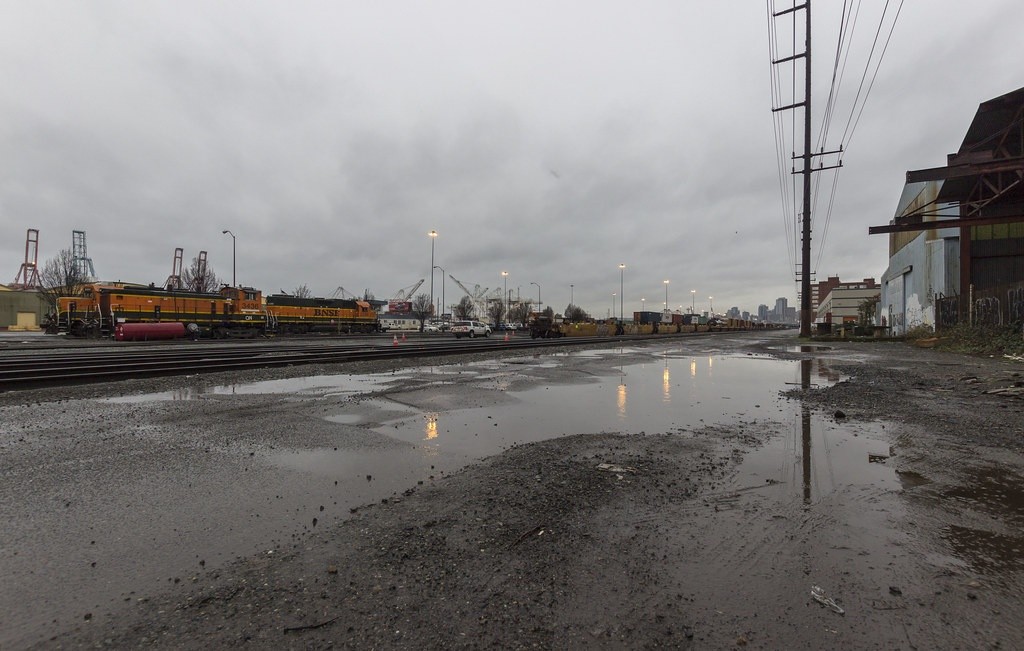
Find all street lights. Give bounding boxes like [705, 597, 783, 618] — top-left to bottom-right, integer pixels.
[642, 299, 645, 311]
[531, 282, 540, 317]
[664, 280, 668, 314]
[680, 306, 682, 313]
[430, 231, 437, 324]
[691, 289, 695, 315]
[502, 272, 507, 323]
[571, 285, 574, 321]
[223, 230, 236, 288]
[450, 306, 457, 326]
[434, 266, 444, 332]
[709, 296, 713, 319]
[612, 293, 616, 316]
[664, 303, 665, 312]
[619, 264, 626, 322]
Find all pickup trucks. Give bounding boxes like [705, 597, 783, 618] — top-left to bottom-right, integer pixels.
[377, 323, 390, 333]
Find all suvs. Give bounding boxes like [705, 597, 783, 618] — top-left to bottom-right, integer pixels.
[450, 321, 492, 338]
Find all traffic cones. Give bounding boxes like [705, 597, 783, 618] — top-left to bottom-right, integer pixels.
[393, 334, 399, 348]
[512, 331, 515, 336]
[504, 332, 509, 341]
[402, 333, 406, 343]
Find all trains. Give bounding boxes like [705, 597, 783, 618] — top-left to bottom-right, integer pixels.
[39, 282, 380, 337]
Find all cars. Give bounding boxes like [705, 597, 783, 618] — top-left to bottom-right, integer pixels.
[418, 324, 439, 332]
[389, 323, 401, 330]
[487, 323, 518, 331]
